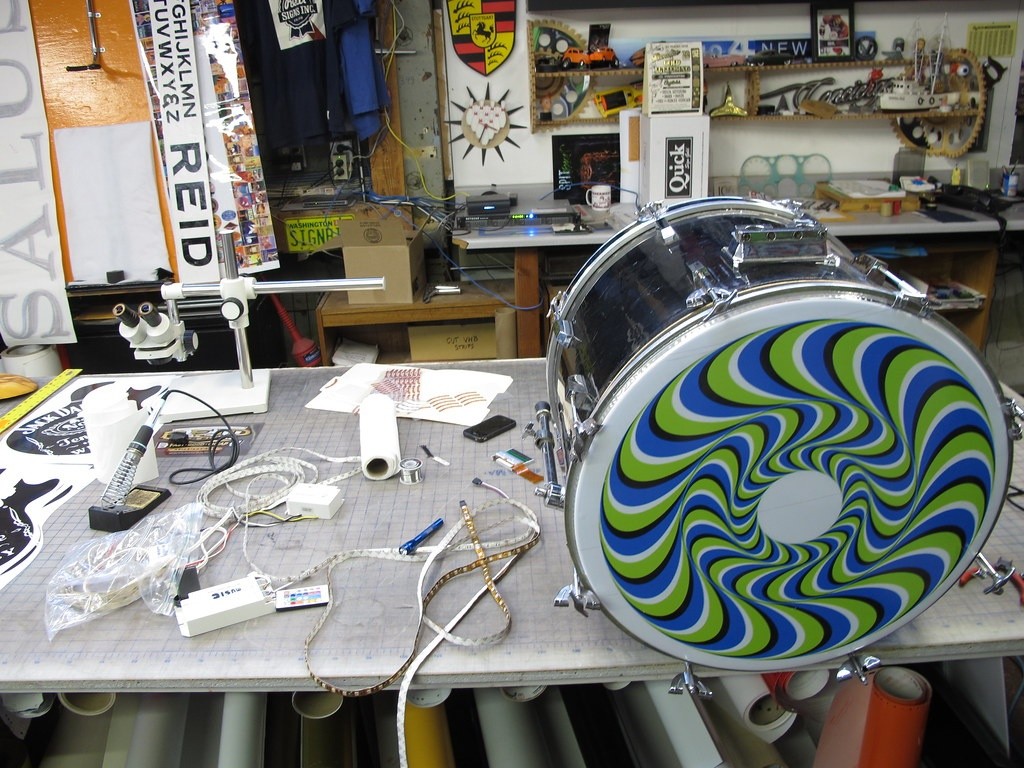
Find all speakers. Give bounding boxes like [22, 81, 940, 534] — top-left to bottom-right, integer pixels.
[963, 159, 990, 189]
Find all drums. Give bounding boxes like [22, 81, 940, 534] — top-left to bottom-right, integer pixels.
[518, 195, 1024, 698]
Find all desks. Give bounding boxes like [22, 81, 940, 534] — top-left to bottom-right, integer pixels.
[315, 278, 515, 367]
[0, 355, 1024, 693]
[452, 163, 1024, 359]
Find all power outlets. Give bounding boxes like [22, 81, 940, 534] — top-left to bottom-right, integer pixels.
[289, 144, 307, 171]
[330, 139, 354, 180]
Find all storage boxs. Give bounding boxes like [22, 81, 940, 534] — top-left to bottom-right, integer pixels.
[638, 112, 710, 209]
[407, 318, 498, 363]
[316, 205, 441, 306]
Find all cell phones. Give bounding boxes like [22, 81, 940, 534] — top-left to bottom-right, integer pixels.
[463, 415, 517, 441]
